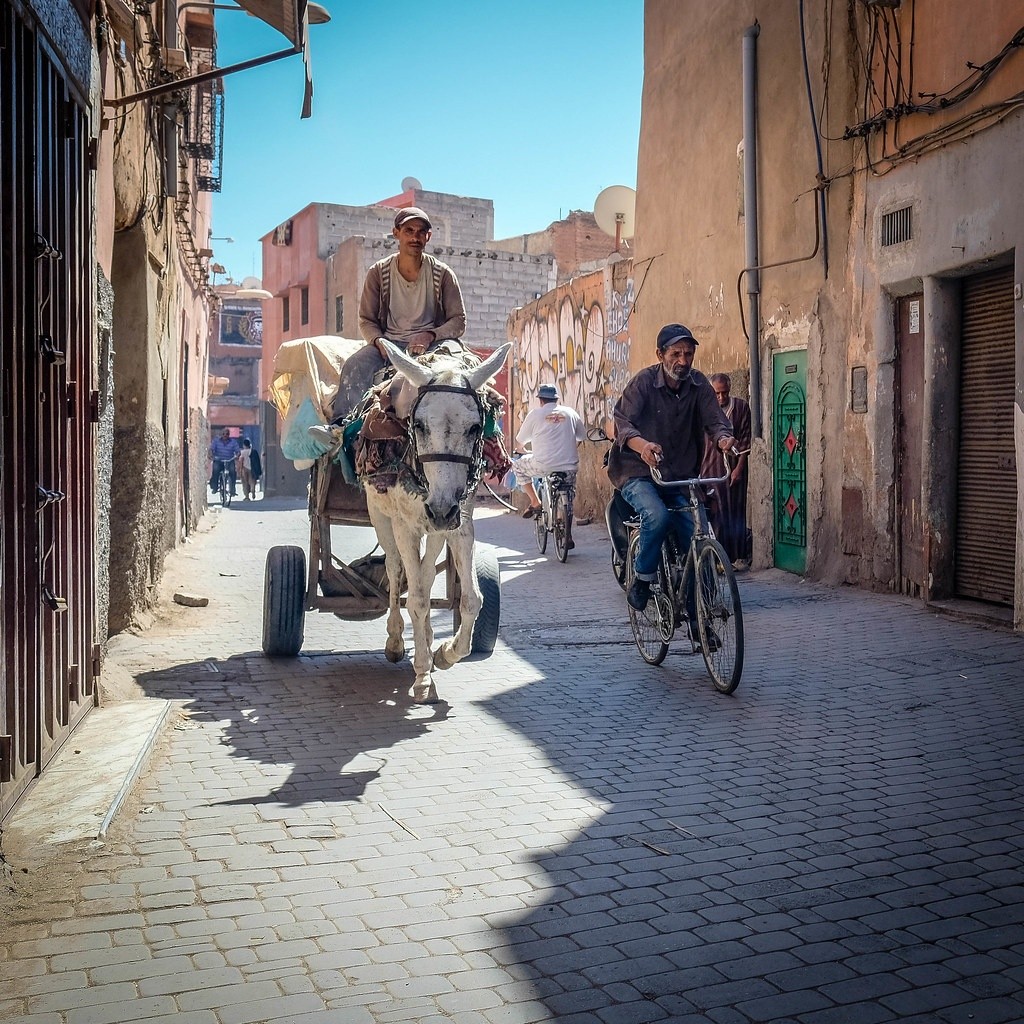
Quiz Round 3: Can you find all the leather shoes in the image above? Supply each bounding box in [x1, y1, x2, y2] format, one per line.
[627, 579, 651, 611]
[686, 627, 725, 650]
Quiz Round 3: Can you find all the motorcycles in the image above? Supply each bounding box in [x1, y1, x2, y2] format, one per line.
[584, 425, 635, 592]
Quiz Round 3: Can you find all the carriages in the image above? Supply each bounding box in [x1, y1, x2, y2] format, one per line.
[257, 338, 513, 705]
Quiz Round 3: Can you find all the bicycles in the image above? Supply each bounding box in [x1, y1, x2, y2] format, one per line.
[212, 455, 240, 508]
[625, 449, 749, 693]
[512, 448, 575, 563]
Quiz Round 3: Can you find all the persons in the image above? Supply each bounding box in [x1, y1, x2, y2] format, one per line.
[238, 439, 262, 500]
[700, 373, 752, 570]
[516, 384, 587, 549]
[607, 324, 739, 648]
[208, 429, 241, 495]
[307, 206, 496, 447]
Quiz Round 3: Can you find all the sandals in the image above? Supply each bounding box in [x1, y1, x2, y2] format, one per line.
[307, 424, 343, 450]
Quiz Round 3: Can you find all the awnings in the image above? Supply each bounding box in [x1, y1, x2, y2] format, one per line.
[105, 0, 313, 119]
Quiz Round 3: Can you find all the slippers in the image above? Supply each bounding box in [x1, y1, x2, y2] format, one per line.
[564, 540, 575, 547]
[522, 503, 542, 518]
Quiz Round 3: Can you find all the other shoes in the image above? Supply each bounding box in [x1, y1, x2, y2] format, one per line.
[242, 498, 251, 501]
[731, 558, 750, 573]
[212, 488, 218, 494]
[229, 492, 238, 496]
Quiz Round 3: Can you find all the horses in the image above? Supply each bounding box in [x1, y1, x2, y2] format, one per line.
[362, 338, 513, 703]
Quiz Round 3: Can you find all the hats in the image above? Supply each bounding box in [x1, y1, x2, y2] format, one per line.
[656, 323, 699, 349]
[536, 384, 561, 399]
[394, 206, 432, 228]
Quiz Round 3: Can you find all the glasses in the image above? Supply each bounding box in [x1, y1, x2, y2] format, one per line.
[222, 432, 229, 436]
